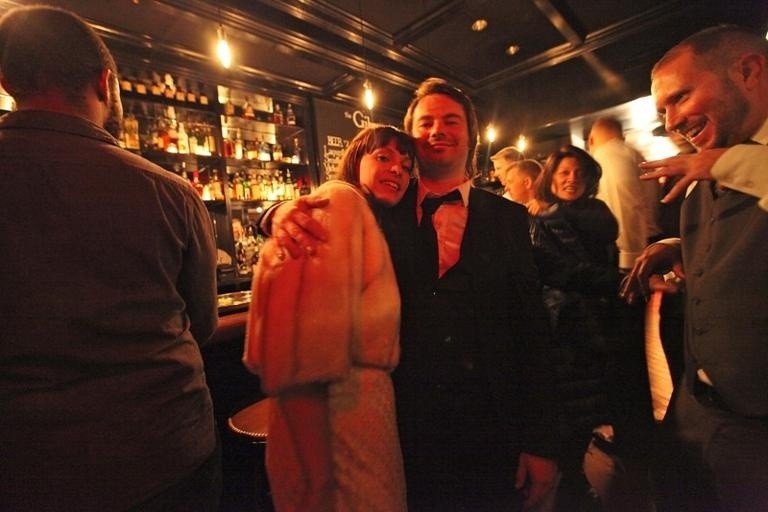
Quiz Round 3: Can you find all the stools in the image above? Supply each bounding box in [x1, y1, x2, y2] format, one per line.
[230, 393, 283, 511]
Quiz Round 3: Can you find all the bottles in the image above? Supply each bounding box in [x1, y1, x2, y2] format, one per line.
[233, 221, 271, 279]
[239, 95, 256, 119]
[271, 102, 283, 125]
[228, 166, 302, 202]
[118, 75, 209, 105]
[118, 108, 217, 157]
[222, 127, 301, 163]
[179, 160, 223, 202]
[284, 102, 297, 126]
[223, 91, 235, 115]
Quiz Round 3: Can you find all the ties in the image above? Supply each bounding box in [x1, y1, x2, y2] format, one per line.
[416, 188, 462, 290]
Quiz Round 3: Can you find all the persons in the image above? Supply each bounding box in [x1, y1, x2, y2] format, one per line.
[488, 144, 526, 206]
[531, 143, 622, 511]
[501, 158, 553, 218]
[255, 75, 563, 512]
[582, 113, 660, 324]
[0, 3, 223, 512]
[240, 123, 416, 512]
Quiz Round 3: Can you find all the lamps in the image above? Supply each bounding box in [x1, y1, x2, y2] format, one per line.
[213, 1, 238, 68]
[359, 1, 378, 110]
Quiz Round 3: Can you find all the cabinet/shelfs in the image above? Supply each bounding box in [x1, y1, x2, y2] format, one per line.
[217, 64, 323, 292]
[95, 34, 237, 297]
